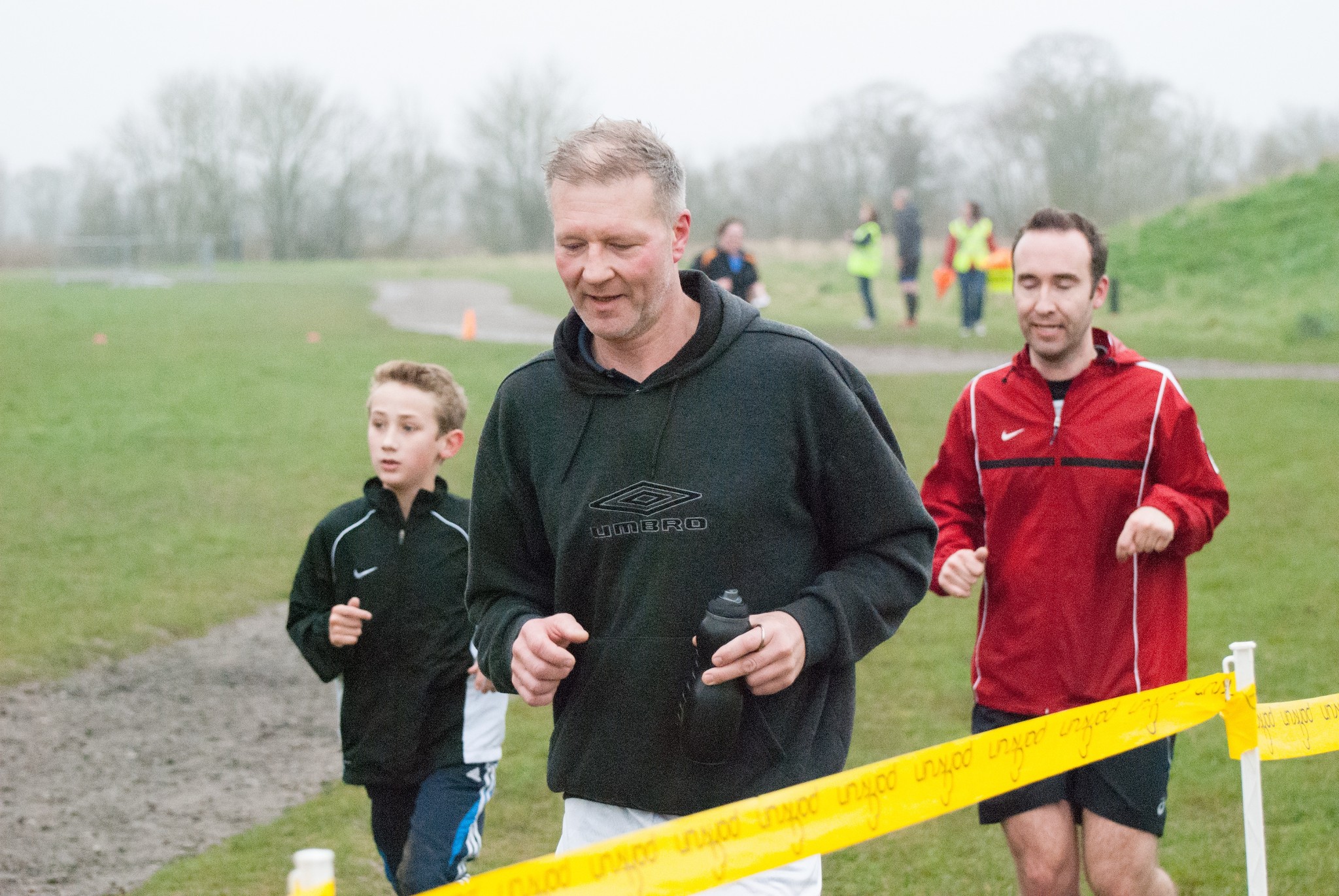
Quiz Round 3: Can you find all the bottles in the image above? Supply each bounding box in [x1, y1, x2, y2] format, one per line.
[678, 588, 754, 765]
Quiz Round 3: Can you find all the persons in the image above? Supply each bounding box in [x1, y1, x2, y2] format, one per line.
[942, 198, 1003, 334]
[918, 206, 1230, 896]
[688, 216, 764, 314]
[842, 204, 881, 328]
[886, 183, 921, 328]
[462, 118, 941, 896]
[284, 360, 515, 895]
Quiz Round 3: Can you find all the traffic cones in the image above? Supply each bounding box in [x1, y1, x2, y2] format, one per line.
[934, 269, 955, 299]
[459, 309, 475, 341]
[982, 247, 1011, 271]
